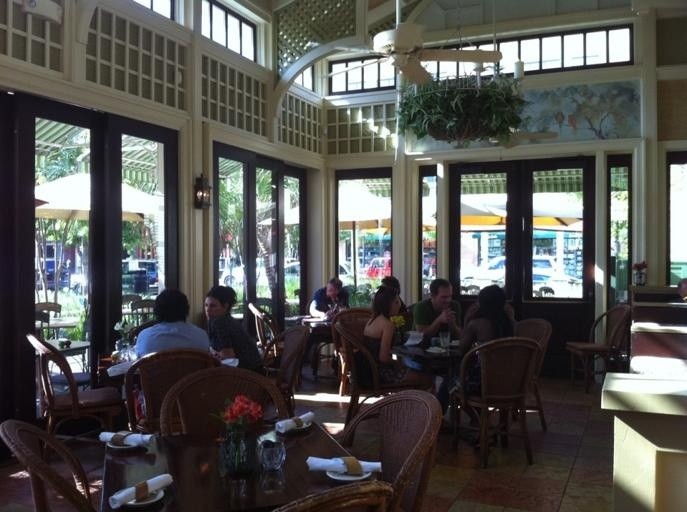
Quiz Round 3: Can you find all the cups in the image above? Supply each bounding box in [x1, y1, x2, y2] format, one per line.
[438, 330, 451, 347]
[256, 440, 288, 470]
[258, 469, 288, 495]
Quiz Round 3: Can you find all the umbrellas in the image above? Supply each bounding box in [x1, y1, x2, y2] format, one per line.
[459, 192, 583, 227]
[35, 173, 157, 223]
[258, 187, 437, 286]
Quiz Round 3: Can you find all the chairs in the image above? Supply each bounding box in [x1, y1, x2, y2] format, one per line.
[566, 304, 631, 393]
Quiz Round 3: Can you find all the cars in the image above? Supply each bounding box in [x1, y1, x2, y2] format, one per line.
[218, 256, 392, 288]
[460, 271, 581, 296]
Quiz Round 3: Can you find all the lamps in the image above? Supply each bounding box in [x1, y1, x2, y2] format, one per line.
[193, 173, 213, 210]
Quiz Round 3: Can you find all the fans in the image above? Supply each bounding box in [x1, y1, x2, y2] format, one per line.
[322, 21, 503, 84]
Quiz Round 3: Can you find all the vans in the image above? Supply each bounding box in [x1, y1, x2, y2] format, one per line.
[70, 259, 158, 295]
[473, 254, 560, 271]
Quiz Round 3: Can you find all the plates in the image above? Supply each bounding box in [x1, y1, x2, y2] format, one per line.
[106, 441, 137, 449]
[325, 471, 373, 482]
[425, 349, 446, 353]
[126, 488, 166, 507]
[295, 421, 312, 430]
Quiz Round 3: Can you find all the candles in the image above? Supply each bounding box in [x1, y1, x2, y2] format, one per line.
[514, 61, 524, 79]
[475, 49, 483, 69]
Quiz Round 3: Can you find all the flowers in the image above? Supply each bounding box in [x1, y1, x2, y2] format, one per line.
[632, 261, 647, 270]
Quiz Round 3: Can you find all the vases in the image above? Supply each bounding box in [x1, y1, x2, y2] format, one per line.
[634, 270, 646, 288]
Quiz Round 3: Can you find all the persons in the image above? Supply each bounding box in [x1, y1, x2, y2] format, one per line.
[449, 285, 517, 448]
[313, 277, 350, 376]
[404, 279, 463, 416]
[359, 286, 434, 393]
[382, 276, 407, 314]
[204, 286, 267, 376]
[134, 289, 209, 419]
